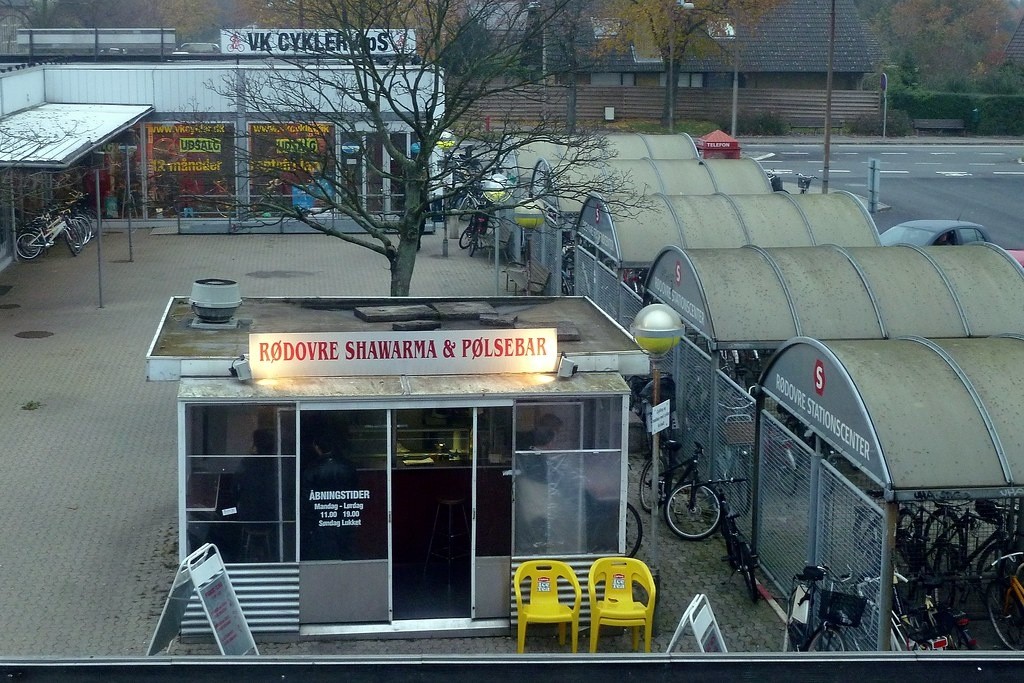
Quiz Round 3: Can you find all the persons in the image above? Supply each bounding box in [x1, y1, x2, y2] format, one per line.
[938, 233, 951, 245]
[220, 429, 362, 561]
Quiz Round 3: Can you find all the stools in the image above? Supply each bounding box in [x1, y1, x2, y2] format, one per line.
[424, 496, 471, 596]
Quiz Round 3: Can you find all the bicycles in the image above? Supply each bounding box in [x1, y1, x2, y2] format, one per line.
[848, 570, 982, 651]
[695, 477, 760, 603]
[693, 339, 847, 493]
[783, 558, 866, 653]
[561, 234, 645, 295]
[16, 189, 98, 260]
[850, 496, 1024, 649]
[459, 201, 488, 257]
[638, 438, 721, 540]
[174, 180, 238, 218]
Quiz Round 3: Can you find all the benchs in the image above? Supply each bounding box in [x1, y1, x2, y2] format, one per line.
[788, 117, 844, 135]
[502, 257, 552, 295]
[481, 222, 511, 262]
[912, 118, 966, 136]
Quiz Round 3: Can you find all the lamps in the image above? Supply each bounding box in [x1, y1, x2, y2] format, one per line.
[558, 360, 578, 377]
[228, 362, 252, 381]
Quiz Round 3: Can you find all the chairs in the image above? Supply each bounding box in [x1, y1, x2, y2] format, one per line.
[588, 557, 657, 654]
[514, 560, 581, 654]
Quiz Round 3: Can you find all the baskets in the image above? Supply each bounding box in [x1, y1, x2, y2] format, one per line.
[818, 587, 867, 628]
[725, 420, 755, 444]
[746, 377, 770, 398]
